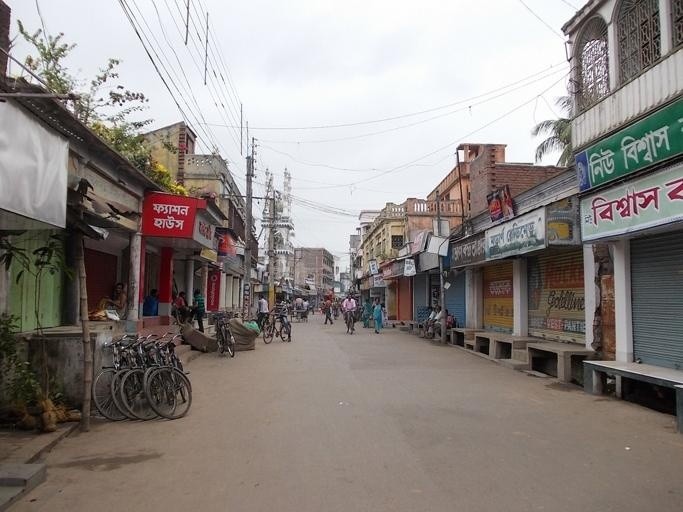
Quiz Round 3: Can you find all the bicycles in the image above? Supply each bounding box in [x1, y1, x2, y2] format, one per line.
[259, 313, 292, 344]
[246, 314, 272, 338]
[342, 308, 357, 334]
[211, 312, 236, 359]
[89, 332, 192, 422]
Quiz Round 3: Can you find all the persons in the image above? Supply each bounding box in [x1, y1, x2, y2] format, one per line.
[143, 287, 205, 333]
[418, 305, 454, 338]
[104, 283, 128, 320]
[257, 294, 269, 329]
[295, 294, 387, 335]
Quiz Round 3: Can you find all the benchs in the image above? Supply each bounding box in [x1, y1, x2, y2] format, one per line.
[406, 319, 682, 429]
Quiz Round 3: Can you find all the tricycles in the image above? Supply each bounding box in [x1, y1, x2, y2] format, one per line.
[291, 306, 308, 323]
